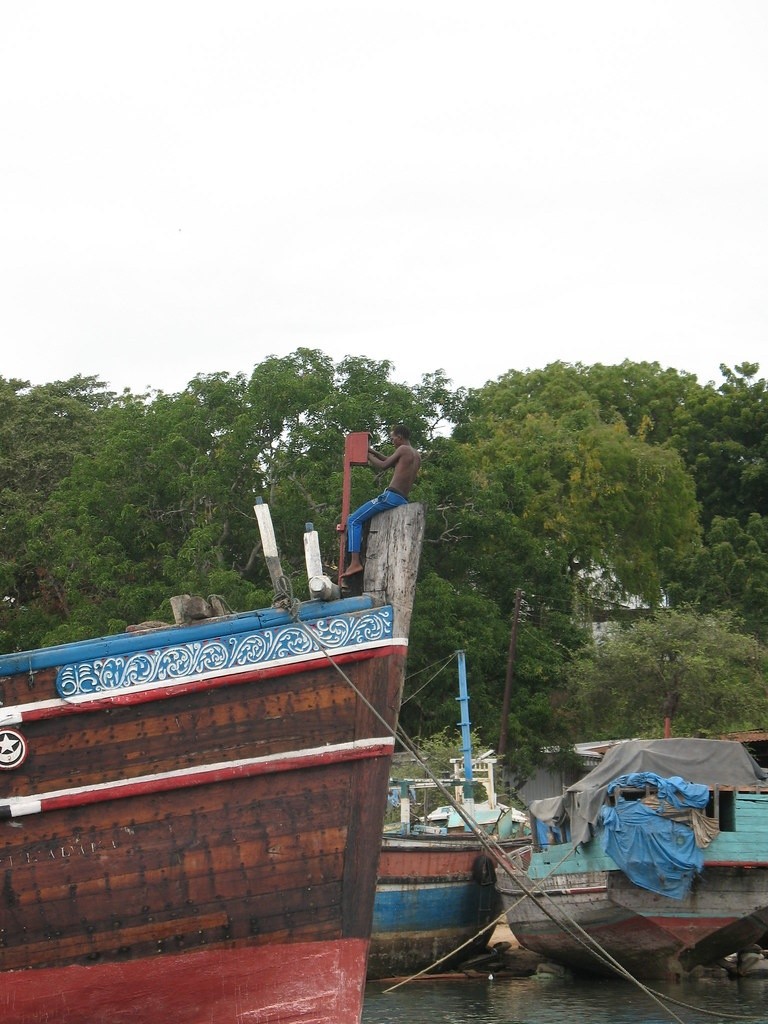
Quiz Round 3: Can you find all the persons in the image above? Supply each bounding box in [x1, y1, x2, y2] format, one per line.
[341, 424, 421, 577]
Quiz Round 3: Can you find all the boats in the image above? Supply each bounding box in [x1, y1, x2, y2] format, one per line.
[0, 596, 409, 1023]
[493, 739, 768, 980]
[366, 652, 556, 979]
